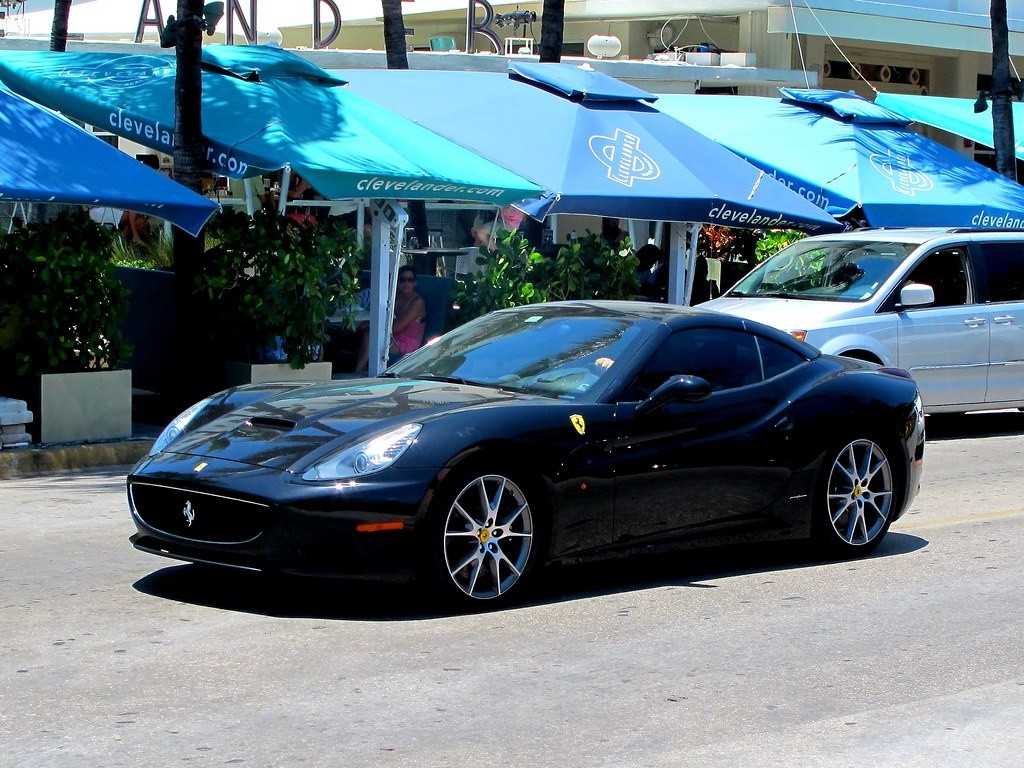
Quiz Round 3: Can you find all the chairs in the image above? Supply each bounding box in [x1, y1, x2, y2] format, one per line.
[691, 337, 737, 393]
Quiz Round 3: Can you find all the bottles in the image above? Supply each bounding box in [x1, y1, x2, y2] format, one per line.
[571, 230, 577, 244]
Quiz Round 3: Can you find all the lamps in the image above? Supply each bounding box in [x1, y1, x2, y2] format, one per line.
[159, 14, 202, 48]
[973, 89, 1013, 114]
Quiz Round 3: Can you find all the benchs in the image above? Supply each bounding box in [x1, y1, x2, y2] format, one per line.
[330, 269, 452, 366]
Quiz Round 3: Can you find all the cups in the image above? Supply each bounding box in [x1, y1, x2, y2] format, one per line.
[274, 182, 279, 189]
[264, 179, 270, 188]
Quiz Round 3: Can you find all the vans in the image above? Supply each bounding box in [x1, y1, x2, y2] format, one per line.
[692, 225, 1024, 414]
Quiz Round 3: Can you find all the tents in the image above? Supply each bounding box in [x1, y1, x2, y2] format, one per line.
[0, 45, 1024, 375]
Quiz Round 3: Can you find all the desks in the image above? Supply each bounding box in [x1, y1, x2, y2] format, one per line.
[325, 309, 397, 323]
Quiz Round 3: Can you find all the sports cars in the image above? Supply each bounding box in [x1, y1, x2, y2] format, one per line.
[124, 301, 927, 610]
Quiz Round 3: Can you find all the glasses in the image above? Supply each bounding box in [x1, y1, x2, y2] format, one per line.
[398, 277, 416, 284]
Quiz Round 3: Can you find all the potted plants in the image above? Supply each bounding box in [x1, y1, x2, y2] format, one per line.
[8, 213, 149, 445]
[202, 211, 332, 384]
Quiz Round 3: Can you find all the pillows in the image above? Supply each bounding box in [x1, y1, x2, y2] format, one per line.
[354, 288, 371, 312]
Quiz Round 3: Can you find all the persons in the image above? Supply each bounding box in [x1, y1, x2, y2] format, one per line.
[476, 208, 524, 252]
[288, 171, 318, 226]
[633, 244, 659, 284]
[88, 206, 148, 248]
[691, 255, 720, 306]
[354, 265, 427, 374]
